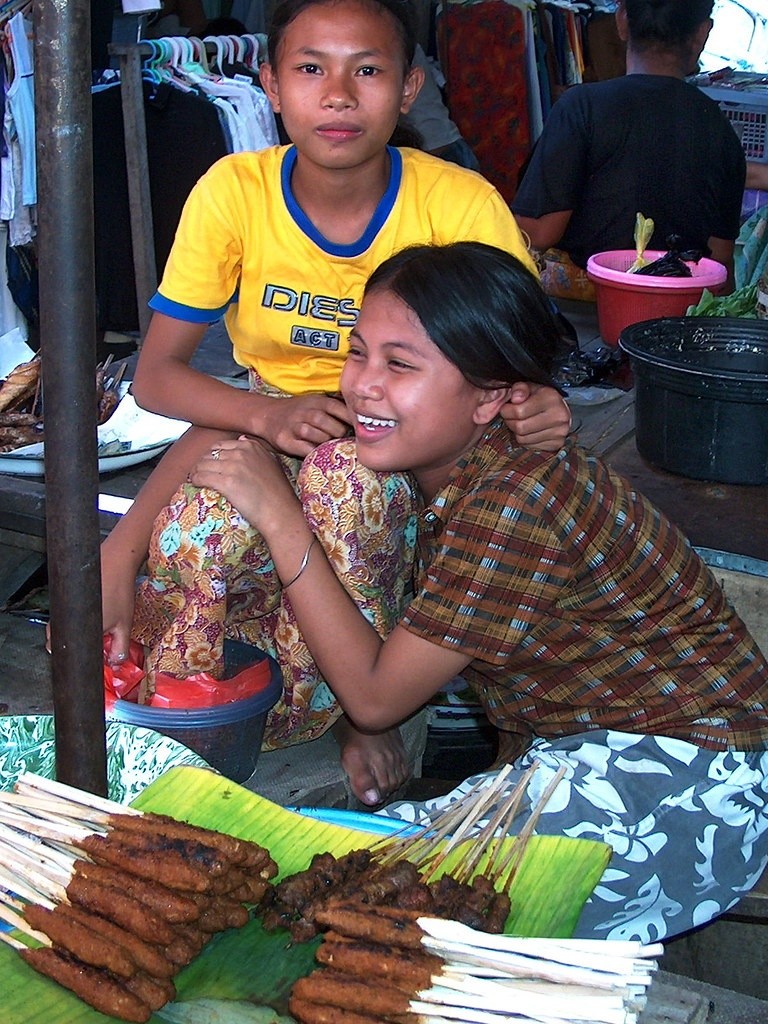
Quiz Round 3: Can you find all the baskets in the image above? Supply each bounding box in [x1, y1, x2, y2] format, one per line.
[105, 636, 283, 787]
[588, 249, 727, 347]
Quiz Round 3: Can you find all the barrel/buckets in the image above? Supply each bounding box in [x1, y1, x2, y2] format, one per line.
[618, 316, 768, 484]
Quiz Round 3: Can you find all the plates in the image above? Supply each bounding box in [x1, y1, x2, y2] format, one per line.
[0, 377, 173, 476]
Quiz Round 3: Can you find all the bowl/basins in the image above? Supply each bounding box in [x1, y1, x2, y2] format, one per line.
[587, 250, 727, 346]
[104, 639, 282, 785]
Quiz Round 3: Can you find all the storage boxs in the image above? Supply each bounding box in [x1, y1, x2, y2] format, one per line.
[685, 70, 768, 165]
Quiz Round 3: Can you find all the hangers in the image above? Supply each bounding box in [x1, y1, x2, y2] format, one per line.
[106, 32, 269, 96]
[0, 0, 33, 56]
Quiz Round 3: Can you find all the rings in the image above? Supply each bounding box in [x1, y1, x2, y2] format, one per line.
[211, 448, 221, 460]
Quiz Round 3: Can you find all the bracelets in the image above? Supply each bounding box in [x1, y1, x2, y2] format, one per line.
[280, 529, 317, 596]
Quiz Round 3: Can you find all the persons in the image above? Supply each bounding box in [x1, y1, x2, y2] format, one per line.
[131, 0, 574, 806]
[45, 231, 768, 943]
[745, 161, 768, 189]
[405, 41, 480, 172]
[511, 0, 746, 263]
[147, 0, 250, 39]
